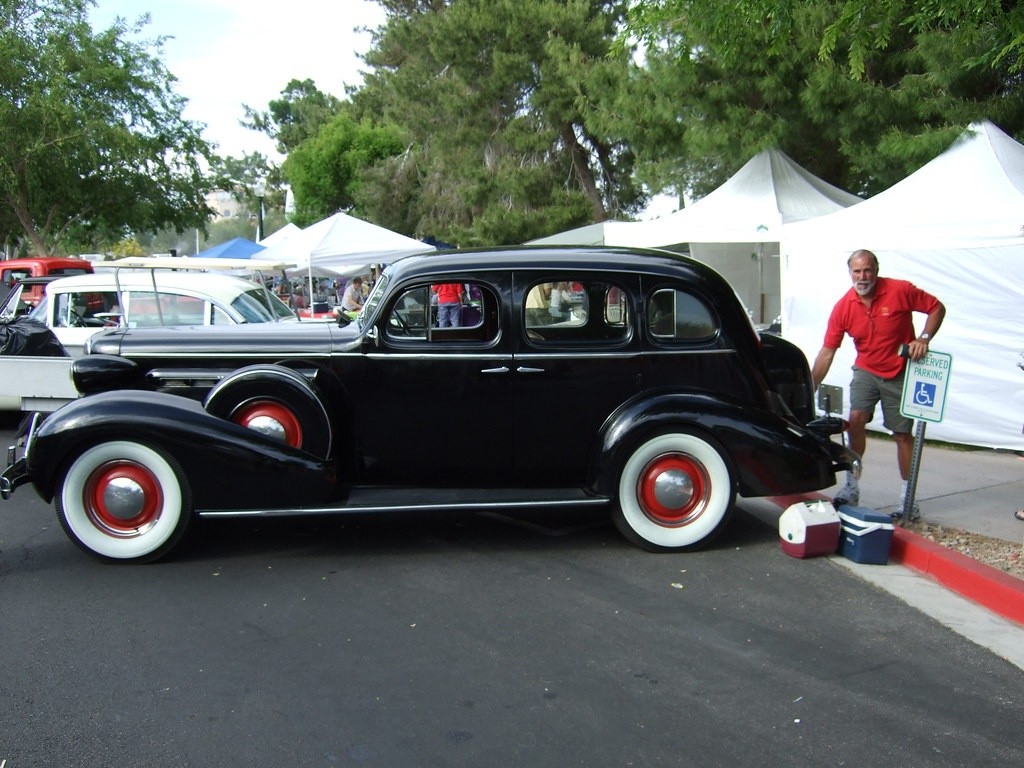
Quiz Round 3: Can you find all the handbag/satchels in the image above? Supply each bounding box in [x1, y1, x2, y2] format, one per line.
[559, 301, 569, 312]
[0, 314, 70, 356]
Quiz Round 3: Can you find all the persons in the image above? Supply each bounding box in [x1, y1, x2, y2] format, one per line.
[103, 305, 122, 327]
[264, 275, 481, 328]
[526, 279, 626, 327]
[812, 249, 946, 523]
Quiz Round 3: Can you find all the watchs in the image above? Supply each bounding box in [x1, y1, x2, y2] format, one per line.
[920, 333, 930, 342]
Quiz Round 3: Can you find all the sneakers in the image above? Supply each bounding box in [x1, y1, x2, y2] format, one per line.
[834, 482, 858, 507]
[890, 503, 921, 521]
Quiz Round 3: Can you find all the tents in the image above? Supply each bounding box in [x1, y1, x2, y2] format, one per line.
[603, 112, 1024, 453]
[187, 212, 457, 318]
[519, 219, 625, 246]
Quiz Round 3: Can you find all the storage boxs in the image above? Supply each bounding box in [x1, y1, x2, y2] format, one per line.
[779, 500, 894, 565]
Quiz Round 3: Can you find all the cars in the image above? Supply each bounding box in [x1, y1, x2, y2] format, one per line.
[0, 243, 861, 567]
[0, 254, 299, 412]
